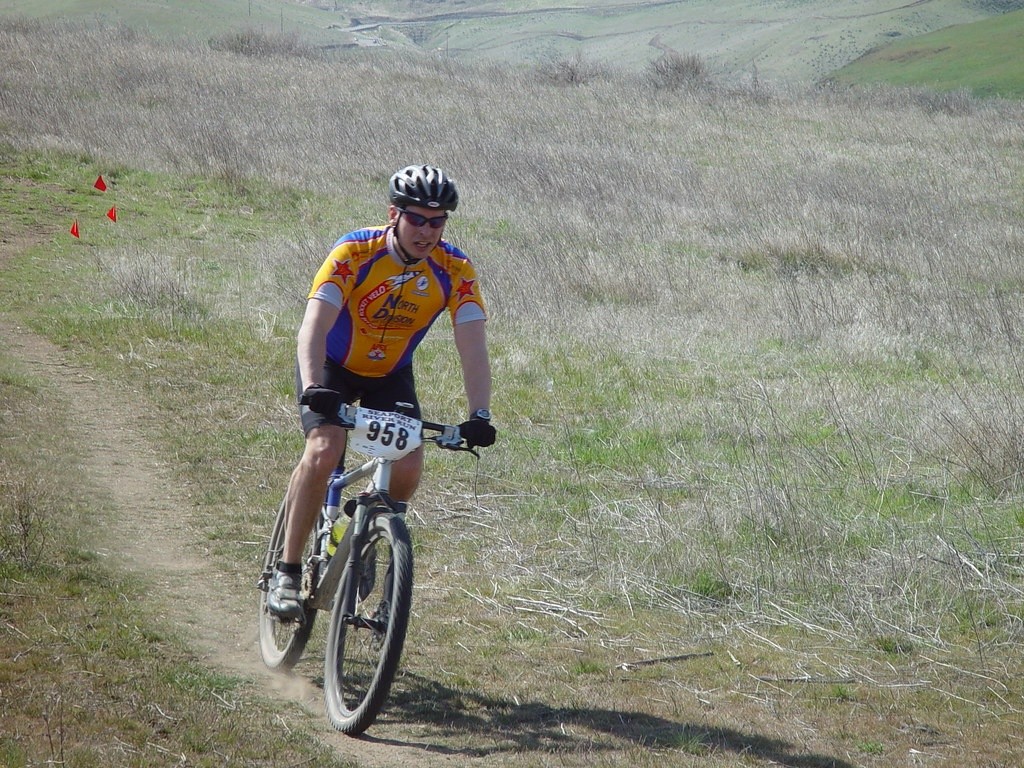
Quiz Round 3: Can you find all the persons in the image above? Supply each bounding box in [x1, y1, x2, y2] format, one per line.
[268, 164, 497, 633]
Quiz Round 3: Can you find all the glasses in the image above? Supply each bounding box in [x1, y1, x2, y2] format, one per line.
[396, 206, 448, 228]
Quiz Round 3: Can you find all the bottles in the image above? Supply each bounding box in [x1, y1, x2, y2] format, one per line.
[325, 499, 356, 556]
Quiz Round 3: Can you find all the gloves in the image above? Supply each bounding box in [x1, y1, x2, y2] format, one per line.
[303, 384, 341, 419]
[457, 420, 495, 448]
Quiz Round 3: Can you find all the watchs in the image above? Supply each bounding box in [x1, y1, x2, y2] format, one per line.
[470, 408, 491, 421]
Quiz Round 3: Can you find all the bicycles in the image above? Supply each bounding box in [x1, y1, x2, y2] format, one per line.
[255, 391, 482, 737]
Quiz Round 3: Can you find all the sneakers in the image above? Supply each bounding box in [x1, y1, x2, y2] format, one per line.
[266, 560, 301, 617]
[359, 548, 377, 601]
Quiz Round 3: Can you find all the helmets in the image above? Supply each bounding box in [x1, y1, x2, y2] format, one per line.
[390, 164, 458, 212]
